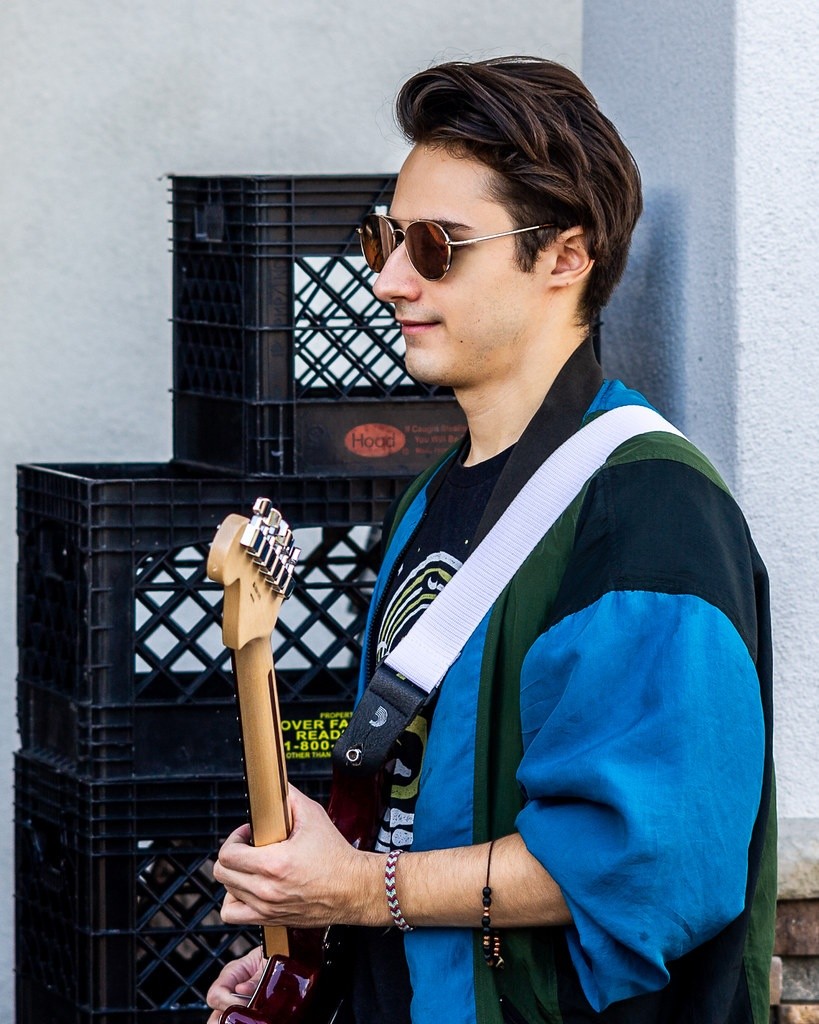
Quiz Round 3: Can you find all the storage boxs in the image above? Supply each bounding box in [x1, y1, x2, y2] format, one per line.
[166, 172, 604, 484]
[10, 751, 412, 1024]
[11, 460, 412, 779]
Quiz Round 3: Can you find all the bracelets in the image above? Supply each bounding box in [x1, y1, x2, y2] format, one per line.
[481, 839, 505, 970]
[385, 849, 415, 934]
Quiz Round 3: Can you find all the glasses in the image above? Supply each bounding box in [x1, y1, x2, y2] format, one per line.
[356, 214, 555, 282]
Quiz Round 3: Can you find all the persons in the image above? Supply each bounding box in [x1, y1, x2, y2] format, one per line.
[205, 54, 778, 1023]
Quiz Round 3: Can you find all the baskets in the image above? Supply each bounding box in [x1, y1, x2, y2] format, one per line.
[18, 460, 418, 781]
[164, 173, 473, 476]
[16, 786, 333, 1024]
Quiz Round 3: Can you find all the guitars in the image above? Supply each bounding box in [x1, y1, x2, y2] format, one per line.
[204, 493, 393, 1024]
